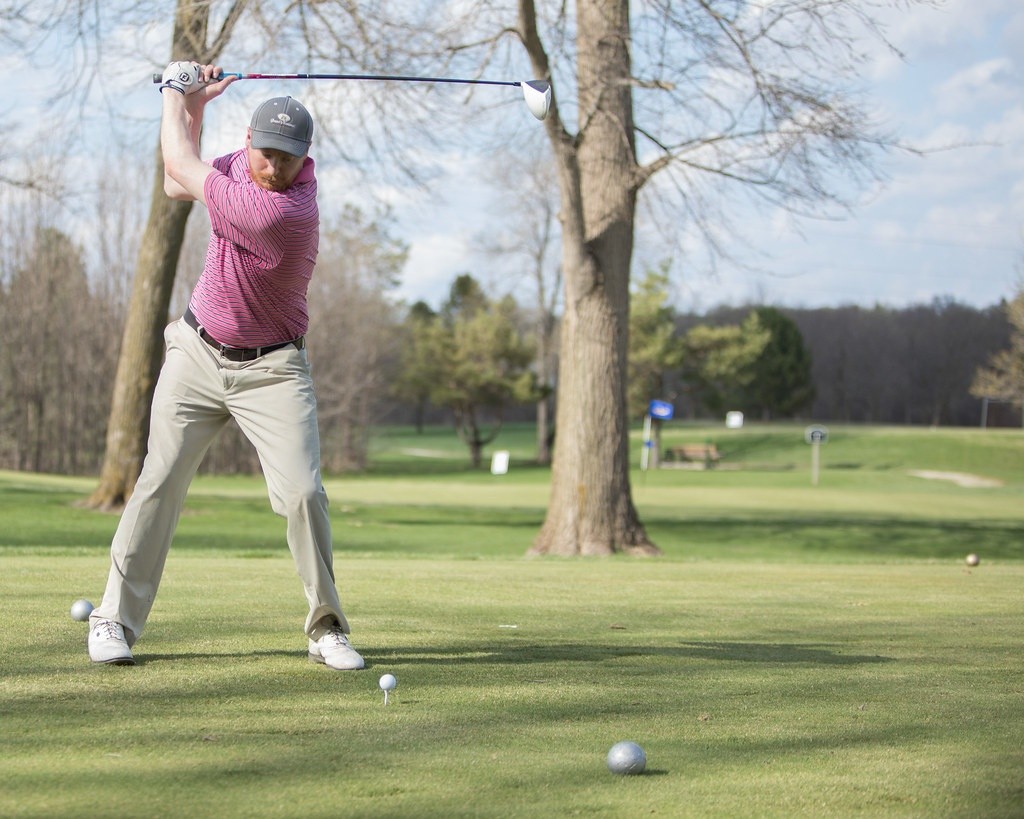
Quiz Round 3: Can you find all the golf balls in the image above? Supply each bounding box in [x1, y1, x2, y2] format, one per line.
[379, 674, 397, 688]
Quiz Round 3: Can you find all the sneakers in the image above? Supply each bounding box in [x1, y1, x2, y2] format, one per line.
[88, 621, 134, 666]
[309, 626, 365, 669]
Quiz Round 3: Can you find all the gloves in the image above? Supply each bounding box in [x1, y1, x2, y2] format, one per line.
[158, 61, 218, 95]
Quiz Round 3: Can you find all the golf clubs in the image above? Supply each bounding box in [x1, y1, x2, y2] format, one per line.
[153, 72, 554, 122]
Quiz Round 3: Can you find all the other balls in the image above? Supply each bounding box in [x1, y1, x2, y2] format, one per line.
[71, 600, 94, 621]
[606, 741, 646, 776]
[966, 553, 979, 566]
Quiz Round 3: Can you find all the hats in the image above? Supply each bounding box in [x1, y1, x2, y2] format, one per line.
[250, 95, 313, 158]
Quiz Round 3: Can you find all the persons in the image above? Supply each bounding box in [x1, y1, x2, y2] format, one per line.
[88, 60, 365, 668]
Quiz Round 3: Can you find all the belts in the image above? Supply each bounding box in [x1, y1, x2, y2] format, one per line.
[183, 306, 305, 362]
